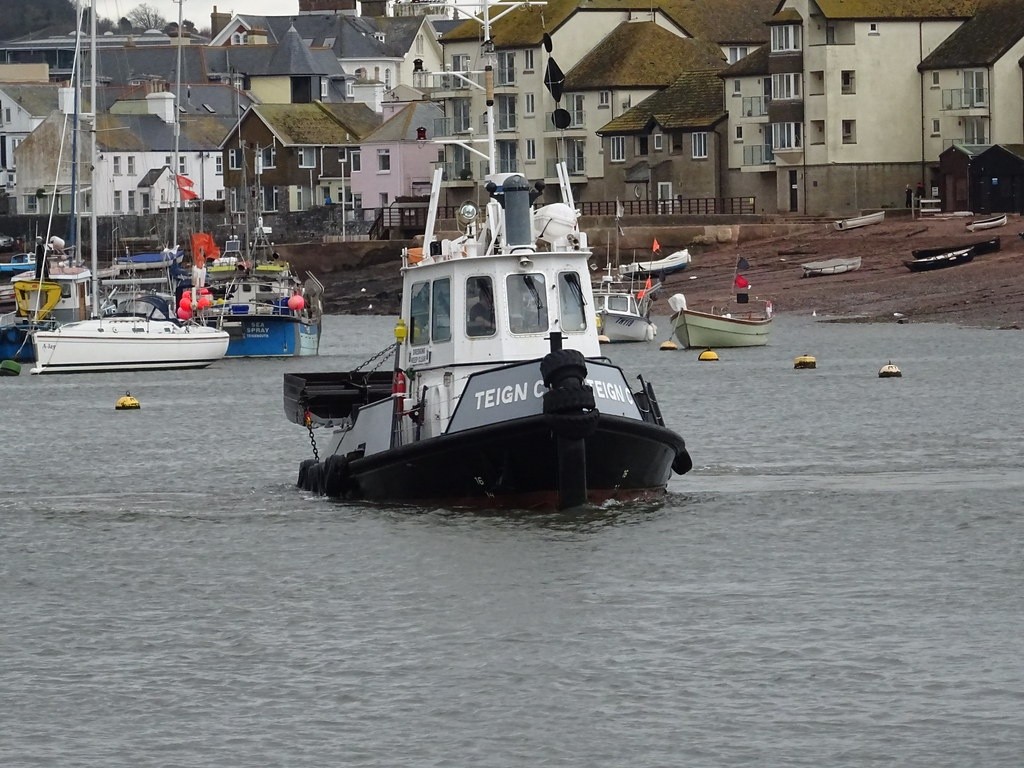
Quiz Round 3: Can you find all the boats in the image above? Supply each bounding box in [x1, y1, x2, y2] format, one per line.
[283, 0, 694, 518]
[800, 256, 863, 279]
[901, 243, 976, 273]
[666, 292, 776, 350]
[833, 210, 886, 231]
[910, 235, 1001, 259]
[965, 212, 1008, 232]
[590, 211, 663, 345]
[618, 248, 692, 281]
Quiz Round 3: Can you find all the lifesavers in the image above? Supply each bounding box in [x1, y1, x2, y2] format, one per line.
[671, 449, 693, 475]
[392, 372, 405, 420]
[296, 453, 346, 496]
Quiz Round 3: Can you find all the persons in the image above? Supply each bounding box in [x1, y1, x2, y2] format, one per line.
[906, 184, 912, 208]
[914, 183, 926, 208]
[471, 287, 495, 328]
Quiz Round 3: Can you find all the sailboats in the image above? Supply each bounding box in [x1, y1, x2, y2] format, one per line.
[0, 0, 326, 379]
